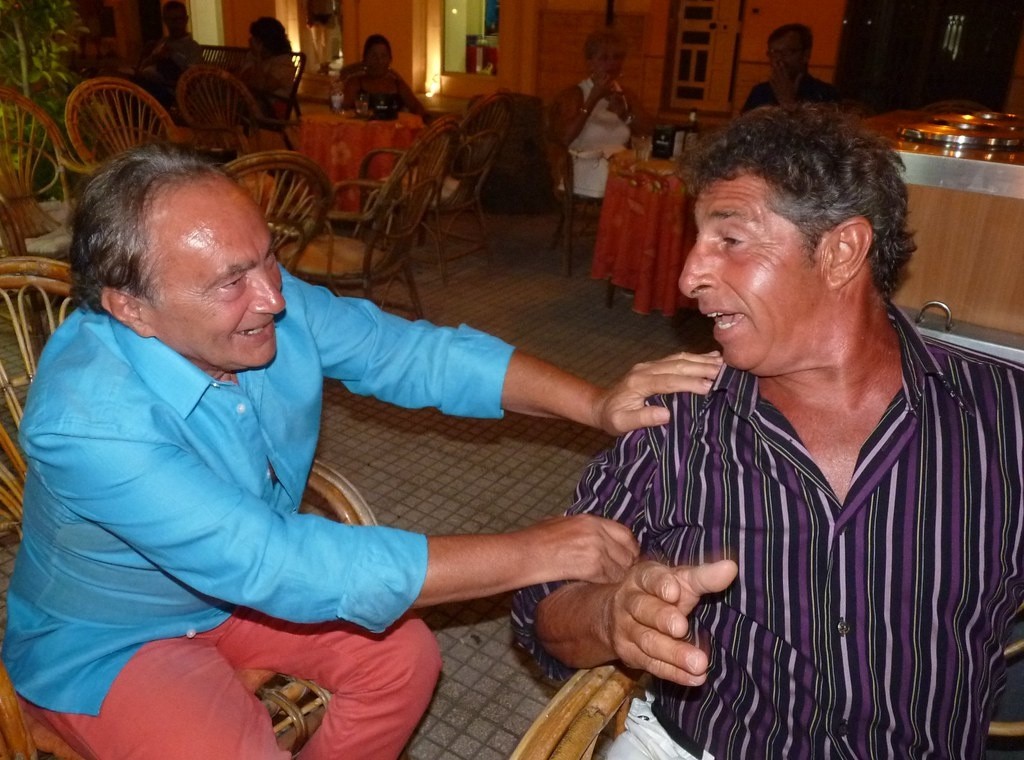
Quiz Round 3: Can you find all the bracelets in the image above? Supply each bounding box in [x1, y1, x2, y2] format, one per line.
[580, 108, 587, 113]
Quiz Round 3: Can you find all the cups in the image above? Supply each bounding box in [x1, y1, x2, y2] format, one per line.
[353, 93, 369, 116]
[631, 133, 652, 163]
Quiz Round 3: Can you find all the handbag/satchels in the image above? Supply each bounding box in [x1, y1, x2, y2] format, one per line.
[556, 148, 618, 199]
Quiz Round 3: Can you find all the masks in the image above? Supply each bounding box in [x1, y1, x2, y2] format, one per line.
[367, 54, 391, 73]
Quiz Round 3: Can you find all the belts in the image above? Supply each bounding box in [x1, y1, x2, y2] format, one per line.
[651, 699, 703, 760]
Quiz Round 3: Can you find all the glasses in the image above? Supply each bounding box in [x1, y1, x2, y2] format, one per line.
[767, 47, 805, 61]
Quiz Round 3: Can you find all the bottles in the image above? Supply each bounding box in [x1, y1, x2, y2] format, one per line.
[683, 107, 699, 152]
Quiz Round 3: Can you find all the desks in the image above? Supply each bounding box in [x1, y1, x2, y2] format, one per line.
[590, 150, 701, 319]
[285, 105, 426, 215]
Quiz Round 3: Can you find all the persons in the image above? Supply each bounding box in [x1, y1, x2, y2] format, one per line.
[329, 34, 433, 126]
[0, 141, 724, 760]
[740, 22, 836, 116]
[511, 105, 1024, 760]
[235, 16, 295, 131]
[553, 30, 635, 206]
[137, 0, 202, 113]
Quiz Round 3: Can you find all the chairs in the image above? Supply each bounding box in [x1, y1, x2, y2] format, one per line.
[1, 45, 1024, 760]
